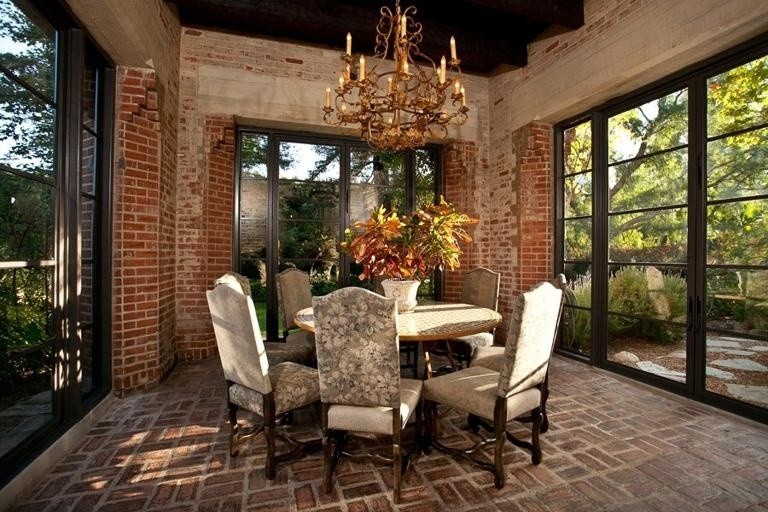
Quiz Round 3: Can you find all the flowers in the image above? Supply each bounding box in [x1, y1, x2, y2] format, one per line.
[340, 193, 480, 281]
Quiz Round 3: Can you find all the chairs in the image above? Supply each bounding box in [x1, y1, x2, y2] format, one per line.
[422, 267, 567, 490]
[203, 266, 322, 480]
[312, 287, 423, 503]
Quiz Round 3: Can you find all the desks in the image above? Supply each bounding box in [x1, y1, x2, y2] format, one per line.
[294, 300, 503, 457]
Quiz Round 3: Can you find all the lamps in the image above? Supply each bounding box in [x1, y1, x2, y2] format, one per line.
[321, 0, 472, 156]
